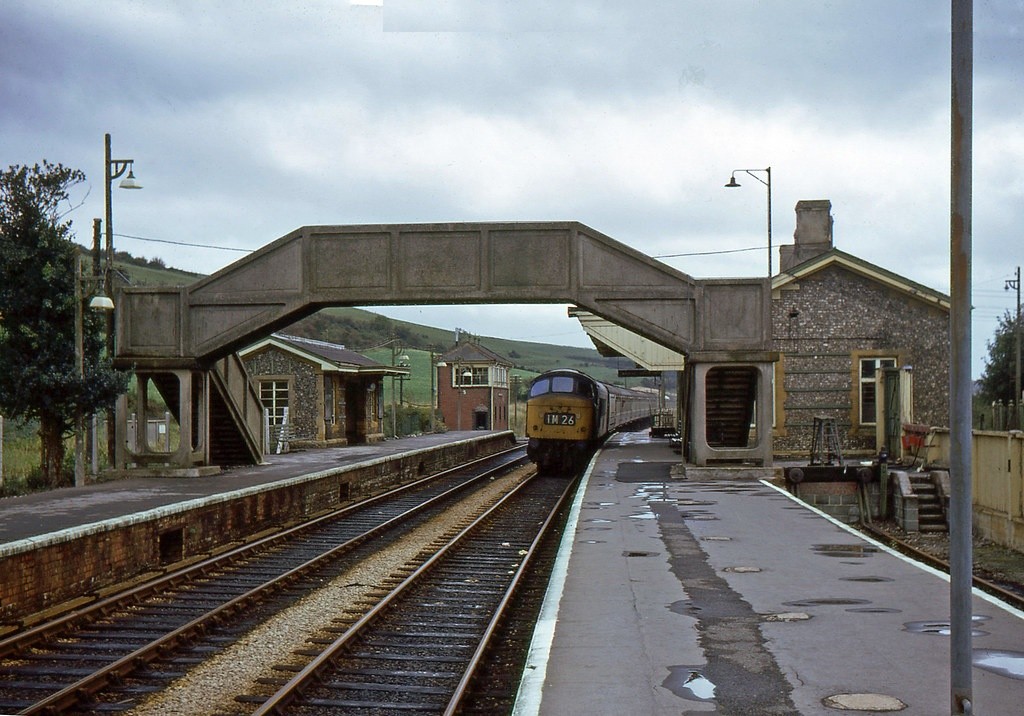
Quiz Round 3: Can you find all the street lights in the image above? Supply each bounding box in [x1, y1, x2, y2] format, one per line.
[1004, 266, 1020, 428]
[74, 252, 114, 487]
[725, 167, 774, 277]
[105, 133, 144, 469]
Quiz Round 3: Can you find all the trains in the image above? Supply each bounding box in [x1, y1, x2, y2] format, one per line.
[525, 368, 670, 477]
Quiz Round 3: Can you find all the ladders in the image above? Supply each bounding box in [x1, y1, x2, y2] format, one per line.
[811, 414, 845, 465]
